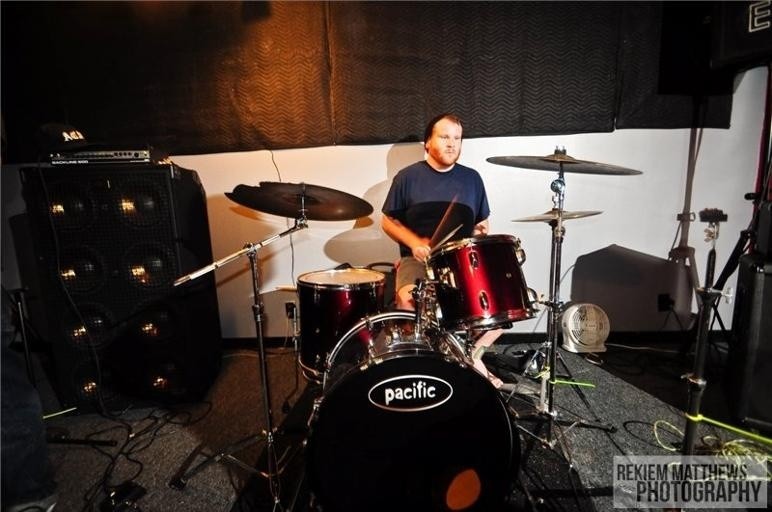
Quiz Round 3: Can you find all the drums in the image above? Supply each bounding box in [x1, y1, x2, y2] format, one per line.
[298, 269, 386, 382]
[301, 312, 522, 512]
[426, 234, 536, 333]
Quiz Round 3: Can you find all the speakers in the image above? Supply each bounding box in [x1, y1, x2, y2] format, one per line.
[721, 253, 772, 438]
[19, 164, 223, 403]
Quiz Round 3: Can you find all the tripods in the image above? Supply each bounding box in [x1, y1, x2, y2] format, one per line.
[170, 251, 310, 512]
[505, 177, 634, 486]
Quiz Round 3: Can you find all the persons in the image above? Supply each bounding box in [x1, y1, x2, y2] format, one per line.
[381, 114, 506, 391]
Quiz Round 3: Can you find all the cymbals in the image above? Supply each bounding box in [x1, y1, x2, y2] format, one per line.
[224, 182, 373, 221]
[511, 212, 601, 222]
[487, 156, 643, 175]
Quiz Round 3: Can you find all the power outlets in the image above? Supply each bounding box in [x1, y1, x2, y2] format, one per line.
[286, 303, 297, 320]
[656, 294, 675, 313]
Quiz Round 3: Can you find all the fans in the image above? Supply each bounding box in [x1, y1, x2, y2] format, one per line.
[562, 301, 611, 353]
